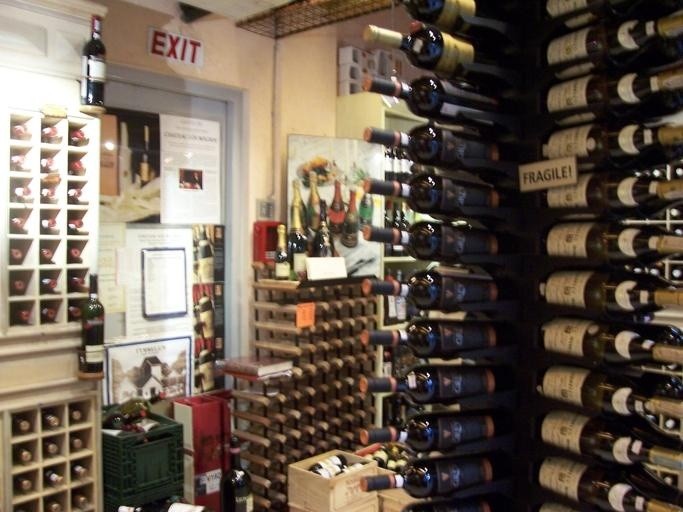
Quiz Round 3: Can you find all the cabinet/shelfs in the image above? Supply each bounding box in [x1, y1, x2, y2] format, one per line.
[220, 252, 383, 509]
[0, 106, 103, 511]
[373, 0, 683, 512]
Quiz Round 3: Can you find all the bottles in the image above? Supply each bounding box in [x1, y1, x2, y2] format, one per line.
[275, 224, 290, 279]
[400, 0, 486, 37]
[541, 219, 683, 266]
[541, 68, 683, 125]
[531, 403, 683, 473]
[359, 408, 494, 453]
[328, 180, 345, 234]
[198, 292, 215, 339]
[199, 338, 216, 392]
[361, 318, 497, 359]
[359, 452, 496, 499]
[530, 450, 683, 512]
[362, 170, 500, 218]
[371, 444, 410, 472]
[361, 74, 502, 131]
[80, 14, 106, 106]
[198, 224, 214, 284]
[102, 411, 146, 429]
[359, 364, 496, 403]
[361, 268, 498, 312]
[540, 269, 682, 314]
[139, 125, 154, 190]
[290, 179, 306, 226]
[81, 273, 104, 373]
[306, 171, 321, 230]
[363, 123, 500, 177]
[289, 203, 307, 273]
[103, 390, 166, 421]
[541, 10, 683, 82]
[219, 436, 254, 512]
[364, 24, 489, 79]
[308, 454, 369, 478]
[539, 170, 683, 214]
[362, 220, 500, 263]
[532, 360, 683, 420]
[541, 123, 683, 162]
[536, 316, 683, 365]
[358, 192, 373, 232]
[314, 200, 334, 258]
[340, 189, 358, 247]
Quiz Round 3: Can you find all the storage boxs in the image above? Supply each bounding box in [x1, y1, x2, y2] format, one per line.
[287, 449, 377, 511]
[102, 410, 183, 508]
[356, 441, 431, 512]
